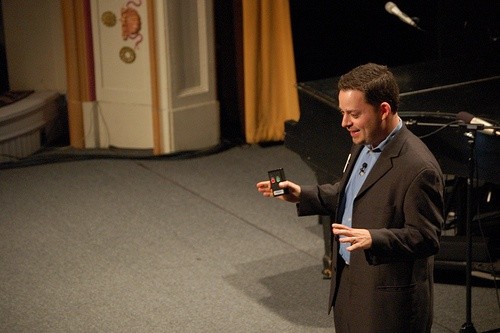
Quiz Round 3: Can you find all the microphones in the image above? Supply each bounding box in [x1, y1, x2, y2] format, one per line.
[385, 1, 422, 32]
[455, 111, 497, 136]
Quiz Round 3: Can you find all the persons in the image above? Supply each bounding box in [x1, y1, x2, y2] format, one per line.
[256, 62, 441, 333]
